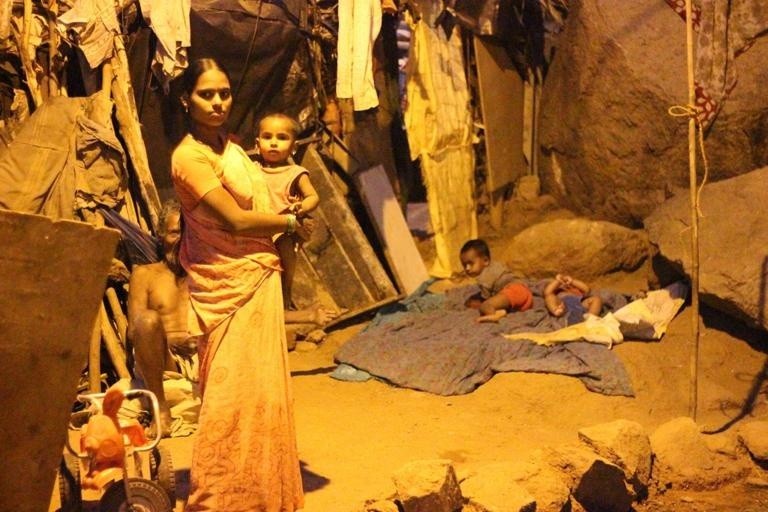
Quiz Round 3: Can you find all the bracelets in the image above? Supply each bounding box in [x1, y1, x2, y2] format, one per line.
[284, 214, 298, 236]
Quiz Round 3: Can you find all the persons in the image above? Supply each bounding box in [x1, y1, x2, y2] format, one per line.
[253, 112, 320, 310]
[281, 306, 336, 327]
[168, 56, 316, 512]
[458, 238, 532, 323]
[542, 274, 603, 327]
[125, 198, 202, 439]
